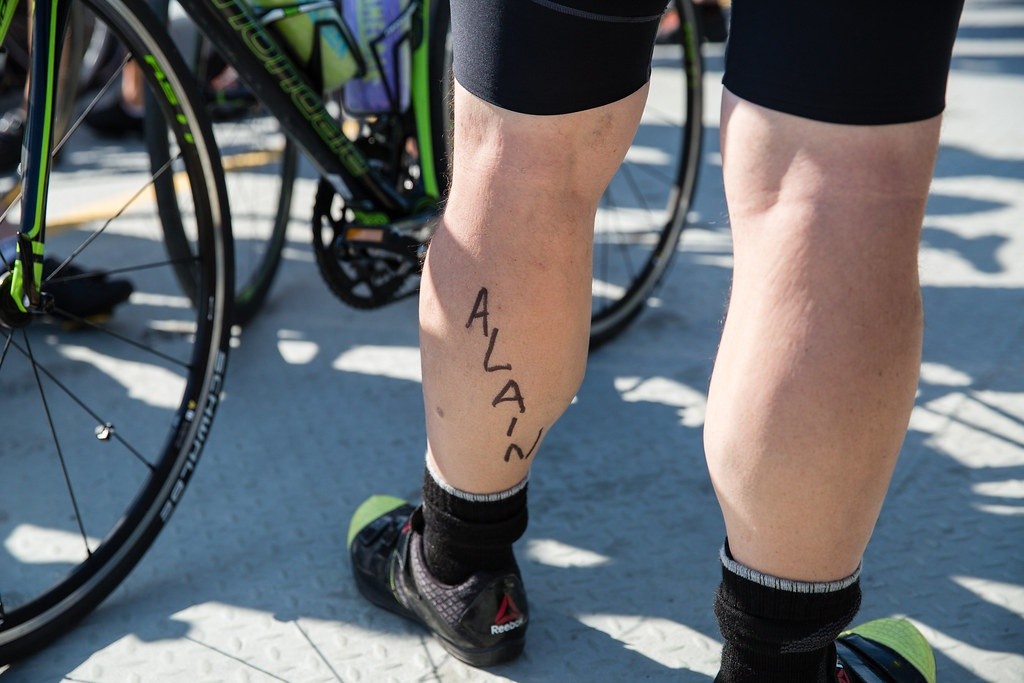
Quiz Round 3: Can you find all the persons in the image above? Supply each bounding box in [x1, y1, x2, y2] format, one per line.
[346, 0, 963, 683]
[0, 1, 260, 319]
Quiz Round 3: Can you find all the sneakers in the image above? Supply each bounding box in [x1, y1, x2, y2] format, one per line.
[831, 615, 936, 683]
[346, 492, 529, 668]
[0, 254, 132, 325]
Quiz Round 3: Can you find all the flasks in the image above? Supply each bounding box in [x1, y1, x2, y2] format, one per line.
[244, 0, 362, 98]
[335, 0, 414, 119]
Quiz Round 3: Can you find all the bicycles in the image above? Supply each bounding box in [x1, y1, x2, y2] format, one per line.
[2, 0, 703, 644]
